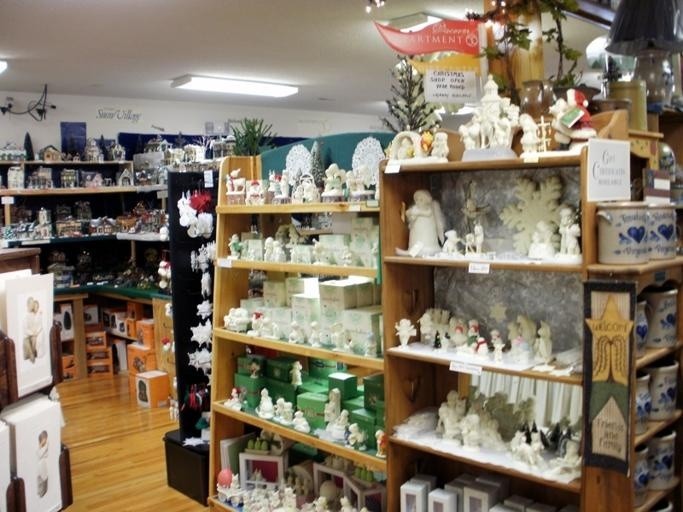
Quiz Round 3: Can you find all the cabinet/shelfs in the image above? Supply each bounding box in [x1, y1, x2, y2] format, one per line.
[378, 108, 630, 512]
[585, 259, 683, 512]
[208, 131, 396, 512]
[164, 166, 219, 498]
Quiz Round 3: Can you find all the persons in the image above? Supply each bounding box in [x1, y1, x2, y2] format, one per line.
[2, 162, 387, 511]
[387, 114, 582, 484]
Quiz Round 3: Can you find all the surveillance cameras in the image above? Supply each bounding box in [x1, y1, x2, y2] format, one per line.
[5, 97, 14, 108]
[36, 101, 56, 111]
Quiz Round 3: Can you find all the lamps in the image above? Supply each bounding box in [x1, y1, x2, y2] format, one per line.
[0, 84, 57, 122]
[387, 15, 443, 33]
[171, 76, 299, 98]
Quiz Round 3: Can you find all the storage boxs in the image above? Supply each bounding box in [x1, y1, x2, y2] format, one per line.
[153, 298, 178, 399]
